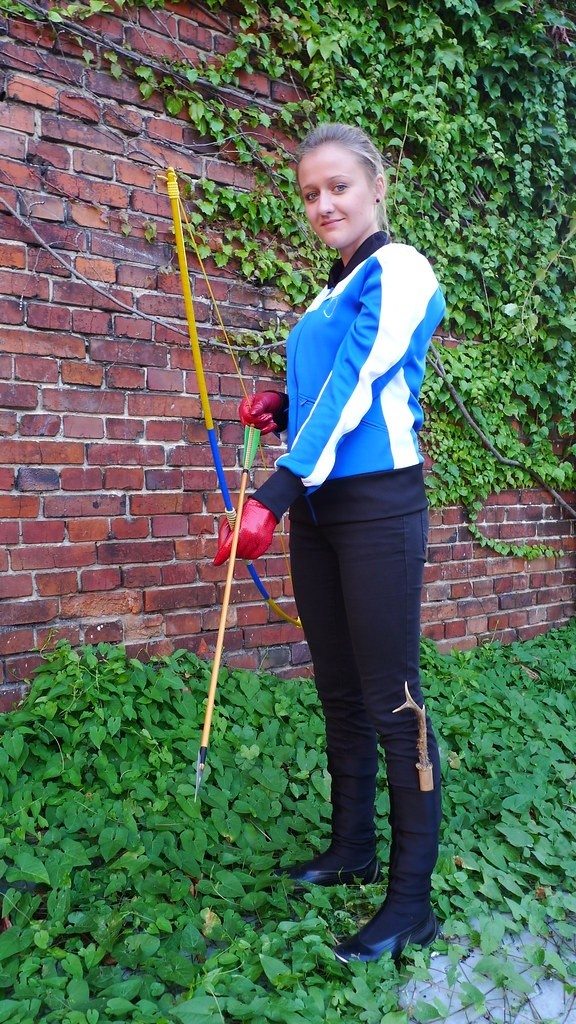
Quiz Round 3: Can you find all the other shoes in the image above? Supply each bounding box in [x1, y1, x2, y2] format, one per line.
[274, 853, 381, 892]
[333, 904, 439, 968]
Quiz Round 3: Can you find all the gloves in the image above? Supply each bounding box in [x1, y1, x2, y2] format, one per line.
[239, 391, 281, 437]
[212, 497, 277, 567]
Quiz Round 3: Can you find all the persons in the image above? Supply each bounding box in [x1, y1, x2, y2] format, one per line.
[212, 123, 446, 969]
